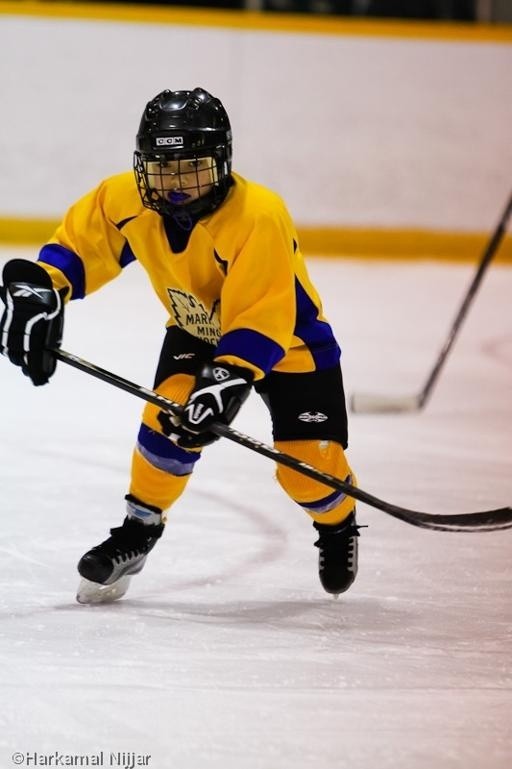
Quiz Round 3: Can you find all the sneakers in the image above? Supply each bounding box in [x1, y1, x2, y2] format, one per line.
[311, 507, 359, 595]
[77, 494, 166, 586]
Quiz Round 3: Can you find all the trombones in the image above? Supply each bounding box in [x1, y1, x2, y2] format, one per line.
[57, 348, 512, 532]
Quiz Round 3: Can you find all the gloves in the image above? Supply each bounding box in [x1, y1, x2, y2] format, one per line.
[0, 253, 70, 387]
[154, 359, 256, 449]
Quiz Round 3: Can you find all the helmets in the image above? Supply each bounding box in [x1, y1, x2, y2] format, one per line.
[131, 86, 233, 223]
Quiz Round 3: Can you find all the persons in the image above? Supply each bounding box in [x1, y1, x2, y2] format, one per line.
[0, 88, 360, 595]
